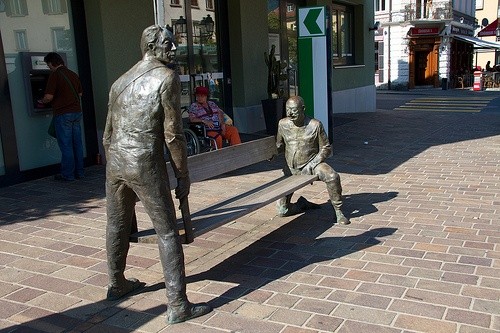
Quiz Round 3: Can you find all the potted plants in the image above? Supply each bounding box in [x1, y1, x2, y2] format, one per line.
[261, 44, 286, 138]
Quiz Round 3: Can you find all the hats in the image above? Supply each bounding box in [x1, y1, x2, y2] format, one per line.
[194, 86, 209, 97]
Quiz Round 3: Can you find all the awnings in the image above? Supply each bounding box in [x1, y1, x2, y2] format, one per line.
[453, 34, 500, 49]
[406, 25, 449, 36]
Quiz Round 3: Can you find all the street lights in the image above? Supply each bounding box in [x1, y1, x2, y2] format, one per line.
[175, 13, 215, 88]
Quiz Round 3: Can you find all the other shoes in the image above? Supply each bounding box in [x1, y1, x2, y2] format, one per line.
[74, 174, 84, 180]
[55, 175, 74, 184]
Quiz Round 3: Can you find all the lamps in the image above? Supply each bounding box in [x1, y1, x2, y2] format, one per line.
[442, 32, 448, 50]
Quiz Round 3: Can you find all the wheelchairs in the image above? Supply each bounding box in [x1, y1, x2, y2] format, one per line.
[180, 105, 222, 157]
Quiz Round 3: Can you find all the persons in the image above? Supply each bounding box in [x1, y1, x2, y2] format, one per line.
[102, 22, 212, 325]
[188, 86, 242, 149]
[485, 60, 491, 71]
[269, 95, 353, 225]
[35, 52, 87, 182]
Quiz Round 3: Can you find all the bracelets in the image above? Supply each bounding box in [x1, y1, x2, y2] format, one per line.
[201, 119, 205, 122]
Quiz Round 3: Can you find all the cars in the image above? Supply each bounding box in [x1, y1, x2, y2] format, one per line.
[182, 89, 189, 95]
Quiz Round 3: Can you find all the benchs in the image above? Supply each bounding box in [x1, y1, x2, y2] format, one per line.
[131, 136, 319, 244]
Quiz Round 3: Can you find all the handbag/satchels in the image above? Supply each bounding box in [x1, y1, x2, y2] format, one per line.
[48, 118, 57, 139]
